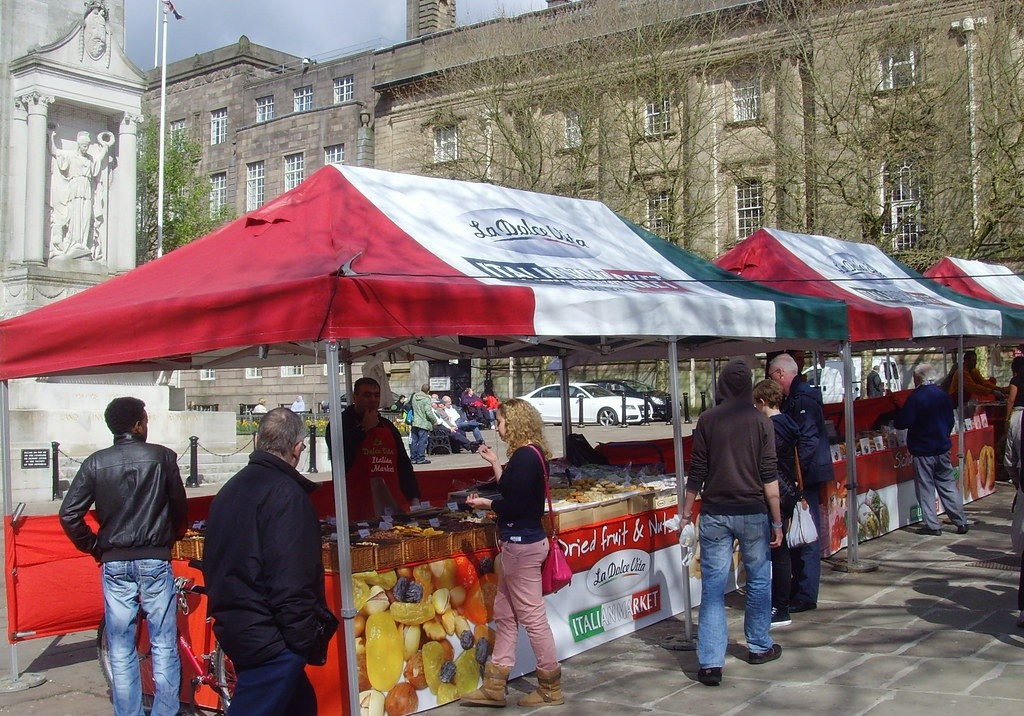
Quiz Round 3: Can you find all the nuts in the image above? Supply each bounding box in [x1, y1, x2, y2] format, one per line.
[347, 558, 499, 716]
[963, 444, 996, 500]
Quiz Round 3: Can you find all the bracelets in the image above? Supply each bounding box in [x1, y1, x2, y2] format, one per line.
[771, 520, 782, 528]
[682, 511, 693, 520]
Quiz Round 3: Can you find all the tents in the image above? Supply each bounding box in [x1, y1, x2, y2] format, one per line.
[886, 256, 1024, 393]
[0, 162, 853, 716]
[546, 227, 1024, 568]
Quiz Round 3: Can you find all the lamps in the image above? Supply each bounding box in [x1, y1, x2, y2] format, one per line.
[302, 58, 310, 68]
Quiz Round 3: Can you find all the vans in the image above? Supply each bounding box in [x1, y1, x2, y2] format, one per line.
[801, 356, 902, 405]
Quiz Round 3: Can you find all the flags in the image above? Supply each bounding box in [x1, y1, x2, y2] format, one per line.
[168, 2, 183, 20]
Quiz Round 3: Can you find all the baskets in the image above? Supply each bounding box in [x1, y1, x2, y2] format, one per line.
[169, 518, 499, 573]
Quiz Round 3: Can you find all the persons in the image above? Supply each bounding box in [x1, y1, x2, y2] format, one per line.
[292, 396, 305, 411]
[395, 383, 499, 464]
[46, 121, 109, 260]
[754, 353, 834, 626]
[254, 398, 266, 412]
[202, 407, 339, 716]
[885, 363, 969, 534]
[188, 401, 195, 410]
[867, 365, 883, 398]
[325, 378, 421, 520]
[1004, 355, 1024, 488]
[949, 350, 1006, 403]
[58, 397, 189, 716]
[680, 358, 782, 682]
[460, 398, 564, 705]
[1004, 410, 1024, 626]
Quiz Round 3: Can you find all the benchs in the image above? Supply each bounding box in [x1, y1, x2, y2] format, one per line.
[427, 429, 453, 457]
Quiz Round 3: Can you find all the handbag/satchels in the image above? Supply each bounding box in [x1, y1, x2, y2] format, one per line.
[405, 409, 413, 425]
[541, 535, 572, 596]
[784, 499, 818, 545]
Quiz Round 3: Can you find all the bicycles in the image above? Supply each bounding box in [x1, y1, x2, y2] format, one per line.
[97, 559, 238, 716]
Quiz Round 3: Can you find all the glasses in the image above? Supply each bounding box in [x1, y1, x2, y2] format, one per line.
[767, 370, 778, 378]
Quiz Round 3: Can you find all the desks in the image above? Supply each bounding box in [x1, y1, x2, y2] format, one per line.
[135, 424, 996, 716]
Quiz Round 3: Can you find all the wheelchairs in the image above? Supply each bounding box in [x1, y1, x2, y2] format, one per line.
[463, 403, 491, 430]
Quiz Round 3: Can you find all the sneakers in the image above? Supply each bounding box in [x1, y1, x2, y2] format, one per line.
[770, 605, 792, 625]
[749, 644, 782, 664]
[698, 667, 722, 683]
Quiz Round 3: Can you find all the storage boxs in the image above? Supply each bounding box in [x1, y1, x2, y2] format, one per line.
[556, 487, 678, 531]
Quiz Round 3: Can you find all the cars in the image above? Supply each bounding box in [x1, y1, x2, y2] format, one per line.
[321, 391, 400, 412]
[587, 378, 682, 422]
[516, 382, 654, 426]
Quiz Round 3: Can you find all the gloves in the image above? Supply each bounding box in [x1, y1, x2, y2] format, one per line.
[90, 544, 103, 559]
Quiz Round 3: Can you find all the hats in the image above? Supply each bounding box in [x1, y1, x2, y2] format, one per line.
[430, 398, 440, 404]
[437, 399, 447, 405]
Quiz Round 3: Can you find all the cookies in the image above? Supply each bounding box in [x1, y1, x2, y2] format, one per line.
[365, 525, 444, 539]
[548, 478, 647, 504]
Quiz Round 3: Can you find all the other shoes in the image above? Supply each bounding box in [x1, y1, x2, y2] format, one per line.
[491, 425, 495, 430]
[1018, 610, 1024, 626]
[959, 523, 969, 533]
[916, 524, 941, 535]
[470, 440, 484, 453]
[789, 598, 817, 612]
[417, 459, 431, 464]
[411, 460, 417, 464]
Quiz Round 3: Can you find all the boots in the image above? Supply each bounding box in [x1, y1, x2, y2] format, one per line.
[518, 663, 565, 706]
[460, 663, 511, 705]
